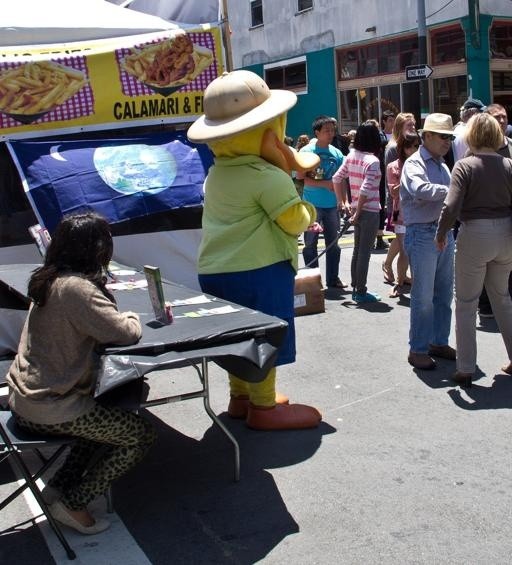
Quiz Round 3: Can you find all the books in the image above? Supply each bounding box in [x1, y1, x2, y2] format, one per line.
[143, 265, 169, 325]
[28, 223, 52, 257]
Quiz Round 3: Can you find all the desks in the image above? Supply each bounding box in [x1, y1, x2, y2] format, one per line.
[0, 242, 289, 514]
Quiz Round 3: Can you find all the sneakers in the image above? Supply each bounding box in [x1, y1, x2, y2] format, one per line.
[352, 289, 380, 304]
[381, 262, 411, 297]
[326, 280, 347, 288]
[408, 351, 437, 369]
[429, 343, 456, 360]
[376, 240, 391, 249]
[41, 484, 110, 535]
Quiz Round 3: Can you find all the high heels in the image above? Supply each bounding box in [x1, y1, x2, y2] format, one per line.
[502, 360, 512, 373]
[450, 371, 474, 388]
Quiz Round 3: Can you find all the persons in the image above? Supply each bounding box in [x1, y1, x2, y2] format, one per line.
[399, 112, 457, 371]
[5, 210, 158, 535]
[387, 127, 421, 297]
[297, 116, 348, 287]
[332, 123, 382, 302]
[435, 112, 511, 388]
[186, 70, 321, 429]
[285, 100, 511, 284]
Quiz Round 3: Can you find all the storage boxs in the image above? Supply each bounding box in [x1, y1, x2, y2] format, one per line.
[294, 266, 326, 320]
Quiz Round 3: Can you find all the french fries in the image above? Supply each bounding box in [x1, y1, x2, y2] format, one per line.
[0, 61, 86, 114]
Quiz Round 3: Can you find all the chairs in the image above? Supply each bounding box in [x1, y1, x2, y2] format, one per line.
[0, 410, 82, 559]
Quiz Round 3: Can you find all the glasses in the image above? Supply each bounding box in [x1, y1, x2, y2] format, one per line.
[437, 133, 456, 141]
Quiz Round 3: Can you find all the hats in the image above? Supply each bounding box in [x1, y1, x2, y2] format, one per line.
[418, 113, 456, 136]
[464, 99, 486, 110]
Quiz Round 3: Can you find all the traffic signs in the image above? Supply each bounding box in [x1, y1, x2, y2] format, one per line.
[404, 63, 435, 82]
